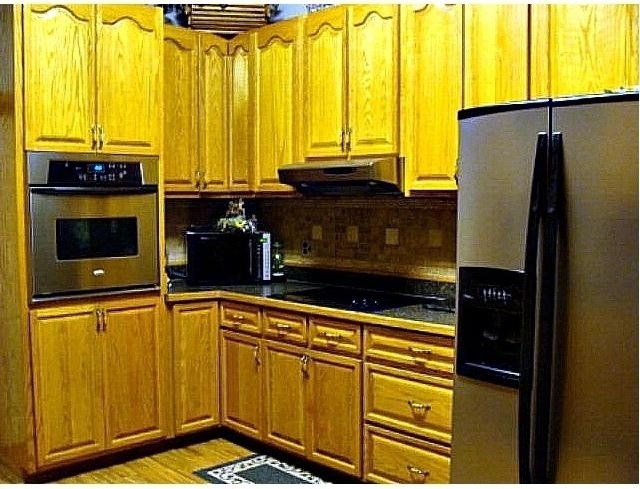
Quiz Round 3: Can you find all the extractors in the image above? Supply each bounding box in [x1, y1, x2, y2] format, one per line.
[276, 158, 405, 197]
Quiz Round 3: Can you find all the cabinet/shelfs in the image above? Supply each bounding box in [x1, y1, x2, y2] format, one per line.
[28, 301, 166, 464]
[163, 24, 233, 191]
[403, 3, 463, 193]
[464, 3, 530, 109]
[171, 301, 220, 437]
[529, 4, 640, 100]
[21, 2, 162, 154]
[221, 299, 263, 441]
[308, 5, 402, 160]
[307, 314, 363, 478]
[362, 324, 454, 485]
[262, 306, 307, 458]
[232, 16, 305, 193]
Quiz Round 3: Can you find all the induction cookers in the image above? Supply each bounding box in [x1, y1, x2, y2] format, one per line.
[270, 284, 434, 314]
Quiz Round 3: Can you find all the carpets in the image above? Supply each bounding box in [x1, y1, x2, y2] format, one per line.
[190, 454, 331, 484]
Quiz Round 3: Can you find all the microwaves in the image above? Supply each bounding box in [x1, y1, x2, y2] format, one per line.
[182, 229, 272, 286]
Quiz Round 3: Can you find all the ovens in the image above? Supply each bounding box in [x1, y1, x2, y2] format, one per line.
[25, 159, 160, 306]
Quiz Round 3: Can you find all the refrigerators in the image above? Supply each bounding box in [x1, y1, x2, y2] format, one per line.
[449, 93, 638, 484]
